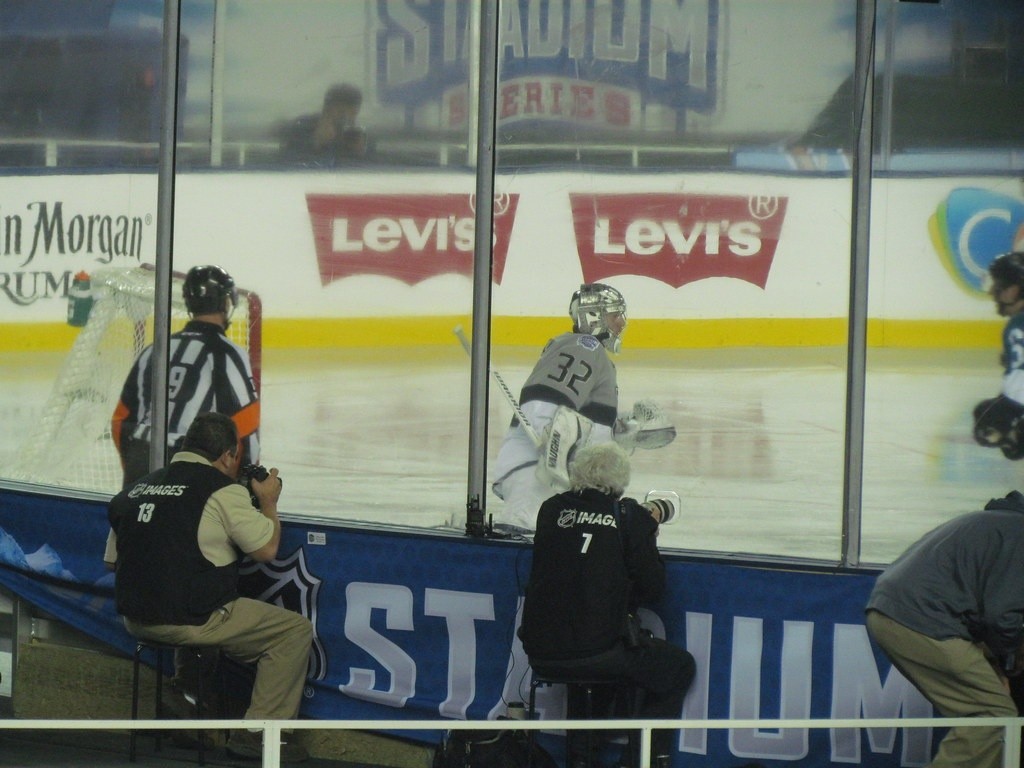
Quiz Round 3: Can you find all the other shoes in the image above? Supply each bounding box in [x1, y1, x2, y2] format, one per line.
[567, 759, 606, 768]
[621, 752, 662, 768]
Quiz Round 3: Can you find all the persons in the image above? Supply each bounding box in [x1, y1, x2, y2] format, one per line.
[863, 489, 1024, 768]
[102, 411, 314, 763]
[515, 441, 697, 768]
[972, 251, 1024, 462]
[491, 283, 677, 541]
[109, 264, 261, 490]
[276, 79, 371, 167]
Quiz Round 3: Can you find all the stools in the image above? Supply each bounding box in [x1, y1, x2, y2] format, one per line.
[128, 639, 229, 768]
[526, 676, 640, 768]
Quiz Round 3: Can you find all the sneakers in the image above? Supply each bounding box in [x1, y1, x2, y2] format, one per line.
[156, 683, 216, 750]
[225, 729, 309, 763]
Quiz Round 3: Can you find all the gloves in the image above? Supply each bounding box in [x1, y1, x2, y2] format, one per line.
[974, 393, 1024, 461]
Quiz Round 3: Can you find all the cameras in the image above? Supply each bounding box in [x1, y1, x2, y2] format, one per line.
[238, 464, 270, 508]
[641, 499, 675, 525]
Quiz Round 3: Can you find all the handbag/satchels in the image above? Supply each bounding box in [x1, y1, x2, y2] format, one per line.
[432, 716, 558, 768]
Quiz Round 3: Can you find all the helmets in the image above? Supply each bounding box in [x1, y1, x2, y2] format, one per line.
[991, 252, 1024, 300]
[183, 265, 239, 331]
[569, 284, 626, 353]
